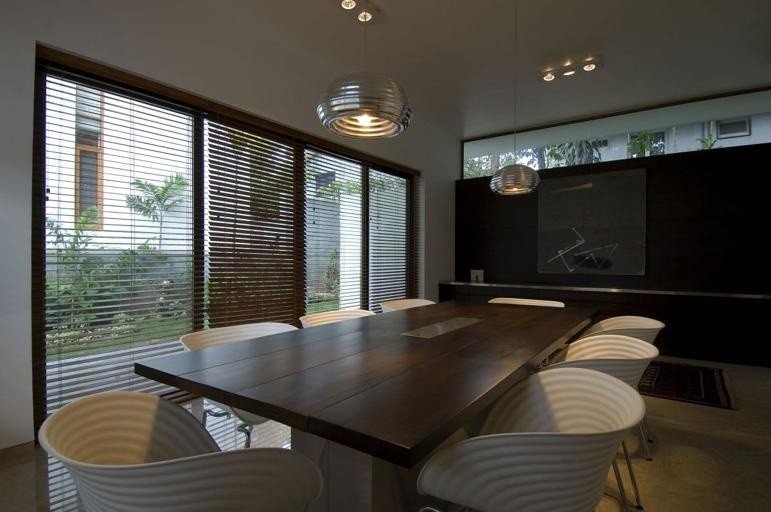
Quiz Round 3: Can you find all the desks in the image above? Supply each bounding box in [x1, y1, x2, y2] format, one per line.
[132, 301, 598, 509]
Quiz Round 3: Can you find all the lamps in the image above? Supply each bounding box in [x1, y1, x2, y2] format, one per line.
[316, 0, 413, 138]
[489, 0, 540, 197]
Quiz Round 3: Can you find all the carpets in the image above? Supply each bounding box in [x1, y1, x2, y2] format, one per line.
[638, 360, 737, 413]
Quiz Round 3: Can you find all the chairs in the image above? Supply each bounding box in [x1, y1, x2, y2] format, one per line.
[178, 322, 300, 448]
[35, 391, 325, 511]
[379, 298, 435, 312]
[489, 297, 566, 308]
[546, 335, 658, 466]
[417, 367, 644, 512]
[577, 314, 665, 352]
[299, 308, 376, 330]
